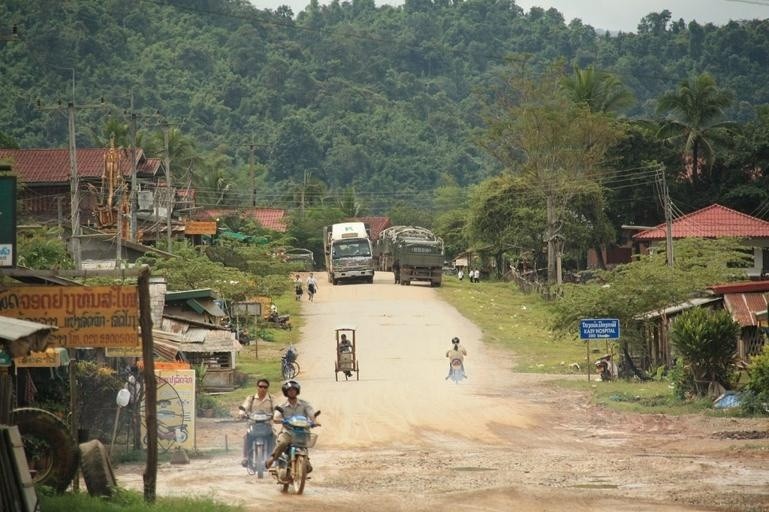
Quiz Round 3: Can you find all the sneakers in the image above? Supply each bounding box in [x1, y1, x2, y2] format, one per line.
[264, 455, 274, 469]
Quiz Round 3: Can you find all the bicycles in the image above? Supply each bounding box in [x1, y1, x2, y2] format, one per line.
[280, 346, 299, 380]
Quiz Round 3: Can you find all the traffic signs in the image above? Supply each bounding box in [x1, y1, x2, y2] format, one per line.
[579, 318, 620, 340]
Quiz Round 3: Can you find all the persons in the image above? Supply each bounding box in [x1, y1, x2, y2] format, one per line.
[338, 334, 354, 353]
[239, 380, 277, 466]
[294, 274, 304, 301]
[469, 268, 474, 283]
[445, 337, 467, 380]
[263, 378, 318, 474]
[306, 271, 319, 302]
[474, 267, 480, 283]
[392, 257, 402, 284]
[457, 269, 465, 281]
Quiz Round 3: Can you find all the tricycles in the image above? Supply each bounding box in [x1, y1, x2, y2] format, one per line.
[334, 329, 359, 382]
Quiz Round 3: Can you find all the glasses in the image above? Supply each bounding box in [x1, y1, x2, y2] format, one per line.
[258, 385, 268, 388]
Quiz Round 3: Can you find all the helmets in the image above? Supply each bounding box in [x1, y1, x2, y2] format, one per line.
[451, 337, 460, 344]
[281, 380, 300, 397]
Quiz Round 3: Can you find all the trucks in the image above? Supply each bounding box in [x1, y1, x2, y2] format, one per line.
[377, 225, 444, 287]
[322, 222, 374, 285]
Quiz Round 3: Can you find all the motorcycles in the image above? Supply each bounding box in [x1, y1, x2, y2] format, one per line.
[238, 405, 275, 479]
[594, 354, 620, 381]
[445, 354, 466, 385]
[269, 406, 321, 493]
